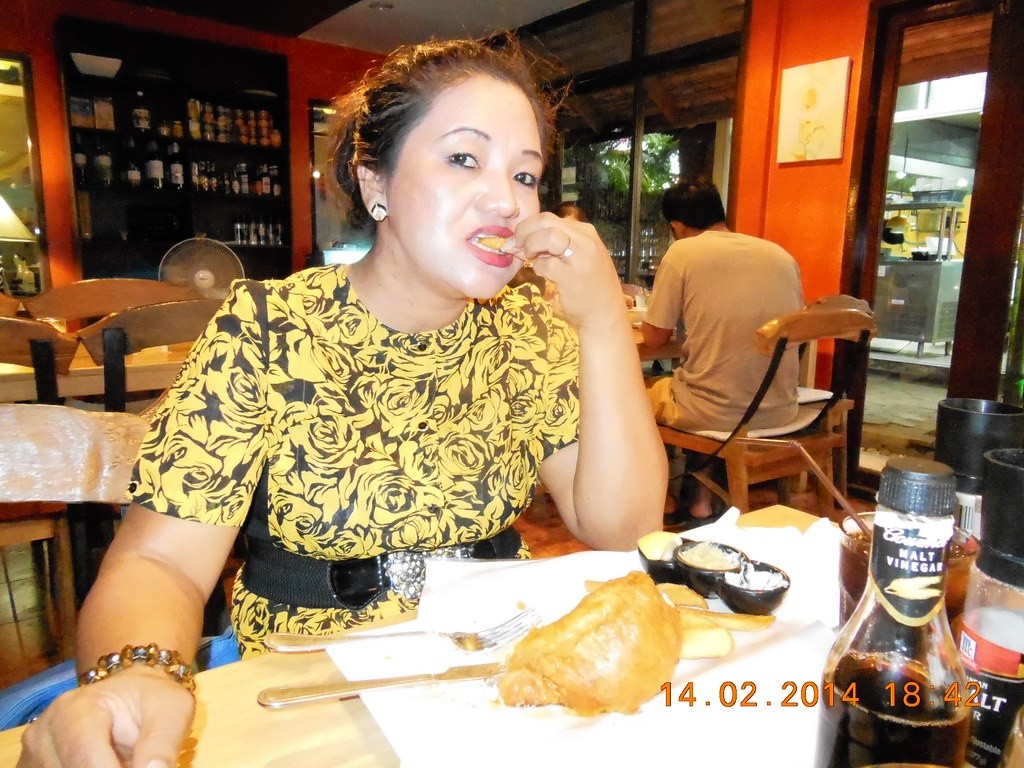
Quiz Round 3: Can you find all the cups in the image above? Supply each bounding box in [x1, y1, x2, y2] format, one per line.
[838, 511, 980, 630]
[634, 294, 649, 310]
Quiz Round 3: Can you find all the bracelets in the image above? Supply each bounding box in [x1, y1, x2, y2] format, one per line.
[77, 643, 197, 695]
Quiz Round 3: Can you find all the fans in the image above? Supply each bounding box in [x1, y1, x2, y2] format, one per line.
[158, 238, 245, 288]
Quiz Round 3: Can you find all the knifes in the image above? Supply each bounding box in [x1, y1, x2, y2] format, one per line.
[258, 663, 505, 708]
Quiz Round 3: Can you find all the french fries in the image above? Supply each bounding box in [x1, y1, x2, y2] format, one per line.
[477, 237, 509, 249]
[584, 580, 775, 660]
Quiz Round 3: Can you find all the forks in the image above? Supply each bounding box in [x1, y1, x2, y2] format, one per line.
[266, 608, 541, 652]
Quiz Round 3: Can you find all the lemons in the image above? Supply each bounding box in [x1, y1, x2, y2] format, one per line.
[636, 530, 682, 561]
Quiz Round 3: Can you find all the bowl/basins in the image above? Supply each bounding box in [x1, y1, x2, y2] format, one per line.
[719, 561, 789, 615]
[673, 542, 748, 599]
[637, 537, 693, 585]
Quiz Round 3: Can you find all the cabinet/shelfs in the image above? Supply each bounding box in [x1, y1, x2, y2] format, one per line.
[54, 13, 292, 280]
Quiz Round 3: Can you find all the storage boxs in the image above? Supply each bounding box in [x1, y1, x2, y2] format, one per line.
[912, 189, 968, 201]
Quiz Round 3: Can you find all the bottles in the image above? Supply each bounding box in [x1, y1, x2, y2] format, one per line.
[71, 132, 89, 184]
[159, 98, 281, 147]
[93, 134, 113, 185]
[169, 141, 183, 189]
[933, 398, 1024, 538]
[815, 456, 971, 767]
[132, 91, 150, 130]
[120, 136, 141, 185]
[143, 140, 164, 188]
[234, 222, 282, 246]
[192, 161, 281, 197]
[956, 447, 1024, 768]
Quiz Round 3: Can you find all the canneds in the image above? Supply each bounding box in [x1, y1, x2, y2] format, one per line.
[160, 98, 280, 148]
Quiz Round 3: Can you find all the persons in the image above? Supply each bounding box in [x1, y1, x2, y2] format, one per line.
[641, 180, 803, 528]
[18, 25, 668, 768]
[552, 204, 634, 309]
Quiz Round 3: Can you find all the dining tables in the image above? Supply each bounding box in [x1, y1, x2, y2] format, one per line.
[534, 327, 826, 496]
[0, 504, 841, 768]
[0, 343, 199, 403]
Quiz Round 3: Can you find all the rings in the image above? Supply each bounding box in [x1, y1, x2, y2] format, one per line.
[556, 236, 573, 260]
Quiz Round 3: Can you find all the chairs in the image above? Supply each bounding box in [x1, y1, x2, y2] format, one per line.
[0, 279, 879, 661]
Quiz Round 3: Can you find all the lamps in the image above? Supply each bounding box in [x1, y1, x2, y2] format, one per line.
[0, 194, 37, 292]
[885, 136, 910, 227]
